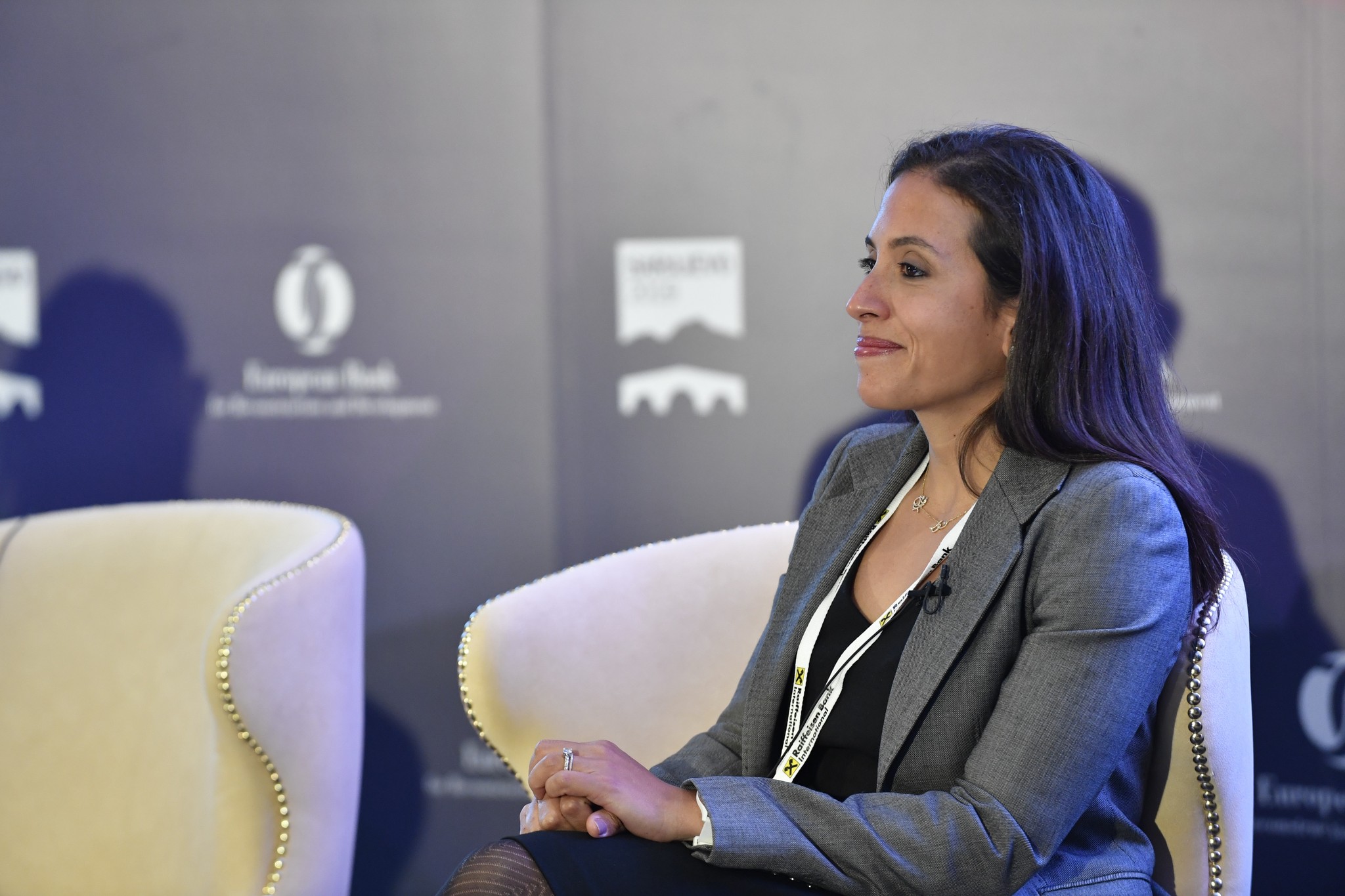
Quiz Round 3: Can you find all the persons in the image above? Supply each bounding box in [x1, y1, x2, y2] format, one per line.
[441, 126, 1223, 896]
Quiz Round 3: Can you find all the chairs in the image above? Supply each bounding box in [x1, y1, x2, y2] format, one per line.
[0, 500, 366, 896]
[458, 521, 1257, 896]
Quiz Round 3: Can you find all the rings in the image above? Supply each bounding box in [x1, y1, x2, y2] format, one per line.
[564, 749, 574, 771]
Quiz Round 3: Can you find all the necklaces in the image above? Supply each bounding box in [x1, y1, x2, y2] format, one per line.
[911, 465, 969, 532]
